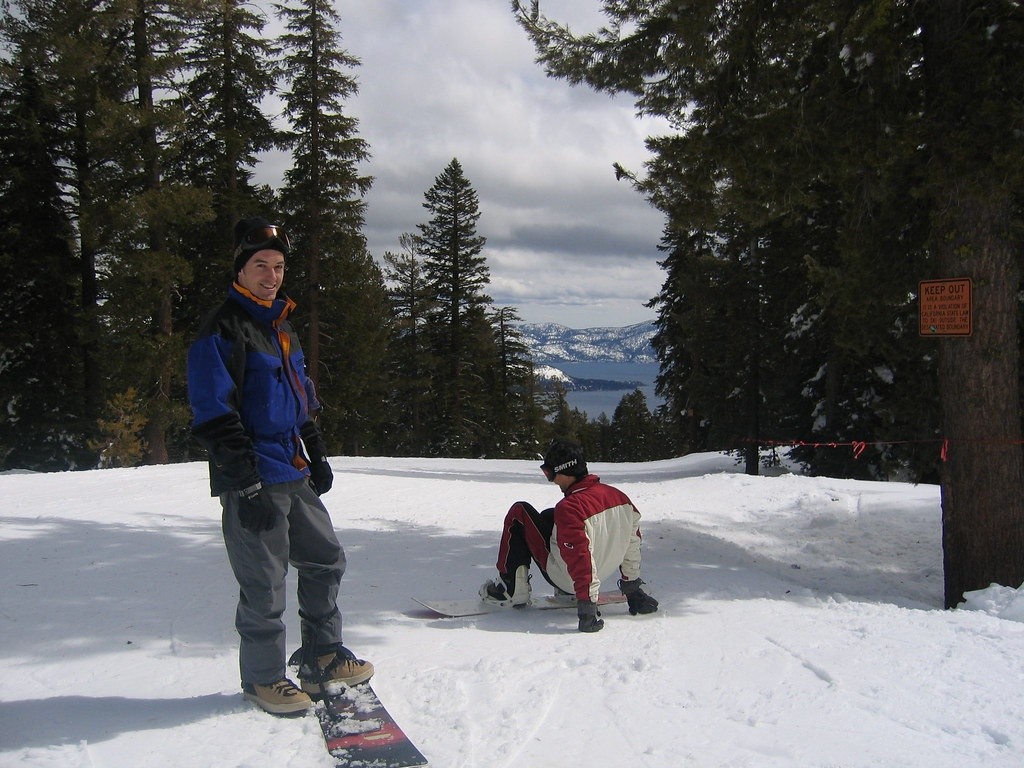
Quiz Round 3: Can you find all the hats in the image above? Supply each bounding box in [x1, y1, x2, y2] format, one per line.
[233, 217, 288, 273]
[543, 440, 588, 477]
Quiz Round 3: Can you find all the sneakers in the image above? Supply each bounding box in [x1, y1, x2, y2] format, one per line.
[297, 642, 374, 694]
[241, 676, 311, 714]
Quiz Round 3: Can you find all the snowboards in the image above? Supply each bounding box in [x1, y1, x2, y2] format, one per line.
[287, 644, 430, 768]
[412, 588, 626, 617]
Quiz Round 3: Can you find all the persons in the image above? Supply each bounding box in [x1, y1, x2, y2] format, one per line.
[189, 220, 375, 713]
[478, 442, 660, 632]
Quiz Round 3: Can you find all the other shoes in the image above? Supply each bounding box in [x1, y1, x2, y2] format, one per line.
[478, 573, 516, 601]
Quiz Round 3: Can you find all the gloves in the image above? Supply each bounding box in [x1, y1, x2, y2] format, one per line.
[235, 473, 274, 535]
[311, 456, 332, 494]
[576, 599, 604, 632]
[617, 577, 658, 615]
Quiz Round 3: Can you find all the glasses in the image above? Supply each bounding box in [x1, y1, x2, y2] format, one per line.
[540, 454, 584, 482]
[233, 225, 293, 262]
